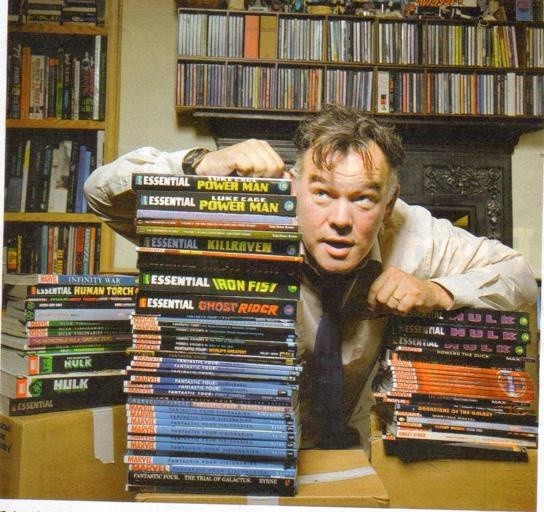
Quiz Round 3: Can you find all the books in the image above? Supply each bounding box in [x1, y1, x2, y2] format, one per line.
[6, 0, 107, 275]
[3, 271, 140, 417]
[374, 300, 538, 458]
[177, 14, 544, 120]
[122, 169, 304, 496]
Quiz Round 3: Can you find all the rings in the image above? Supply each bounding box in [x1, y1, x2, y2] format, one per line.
[392, 294, 402, 303]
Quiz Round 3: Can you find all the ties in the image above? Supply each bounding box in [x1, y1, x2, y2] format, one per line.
[302, 260, 364, 450]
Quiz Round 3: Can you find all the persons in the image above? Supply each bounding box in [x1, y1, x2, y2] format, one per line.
[80, 102, 538, 451]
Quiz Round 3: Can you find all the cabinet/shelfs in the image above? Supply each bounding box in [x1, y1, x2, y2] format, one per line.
[175, 7, 544, 123]
[3, 0, 124, 275]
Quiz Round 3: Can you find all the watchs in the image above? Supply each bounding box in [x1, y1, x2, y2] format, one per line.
[181, 146, 212, 176]
